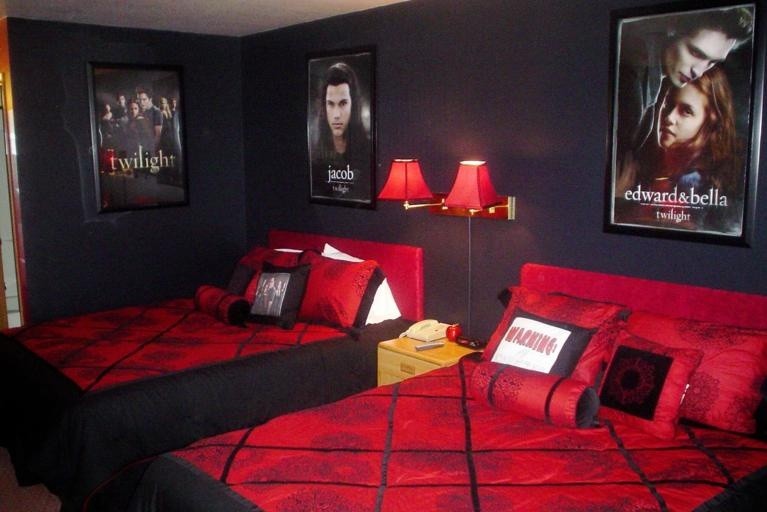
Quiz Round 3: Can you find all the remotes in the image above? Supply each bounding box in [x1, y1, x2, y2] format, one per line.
[416, 341, 445, 351]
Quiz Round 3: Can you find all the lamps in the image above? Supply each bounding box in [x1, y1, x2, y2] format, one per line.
[377, 154, 510, 219]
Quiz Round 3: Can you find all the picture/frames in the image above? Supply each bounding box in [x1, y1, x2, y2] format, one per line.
[83, 56, 192, 215]
[304, 42, 378, 209]
[602, 3, 765, 249]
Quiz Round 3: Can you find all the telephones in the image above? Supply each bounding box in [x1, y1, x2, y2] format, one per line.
[407, 319, 450, 342]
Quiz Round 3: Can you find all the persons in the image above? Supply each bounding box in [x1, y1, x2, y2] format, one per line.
[252, 272, 289, 319]
[312, 63, 371, 201]
[639, 66, 744, 237]
[614, 5, 756, 194]
[92, 82, 183, 188]
